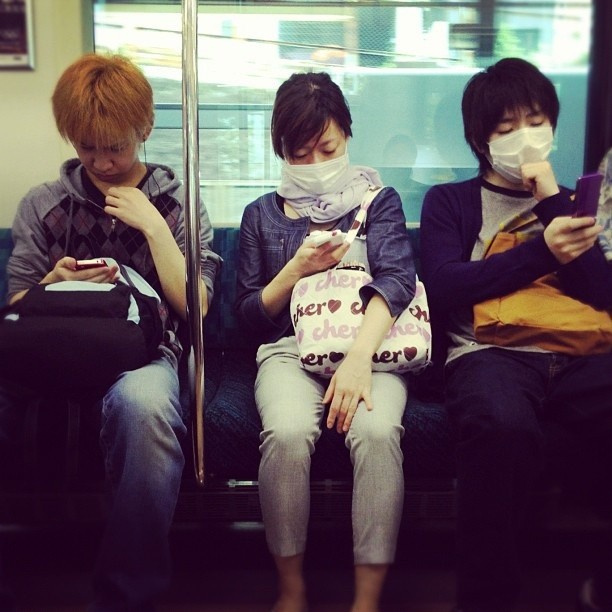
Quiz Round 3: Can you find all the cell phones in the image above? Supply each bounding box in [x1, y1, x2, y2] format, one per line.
[76, 258, 109, 272]
[571, 172, 604, 229]
[312, 228, 344, 253]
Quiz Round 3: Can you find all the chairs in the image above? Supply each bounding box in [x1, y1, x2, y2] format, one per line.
[201, 227, 427, 537]
[0, 225, 201, 538]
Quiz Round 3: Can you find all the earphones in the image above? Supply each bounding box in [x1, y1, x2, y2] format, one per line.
[141, 127, 148, 140]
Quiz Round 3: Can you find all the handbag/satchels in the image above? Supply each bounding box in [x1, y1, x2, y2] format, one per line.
[285, 184, 435, 375]
[472, 192, 612, 366]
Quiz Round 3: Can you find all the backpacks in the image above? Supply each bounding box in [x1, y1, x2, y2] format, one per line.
[0, 258, 169, 398]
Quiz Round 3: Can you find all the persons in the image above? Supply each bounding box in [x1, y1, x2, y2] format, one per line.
[231, 71, 417, 612]
[3, 55, 225, 611]
[419, 56, 612, 611]
